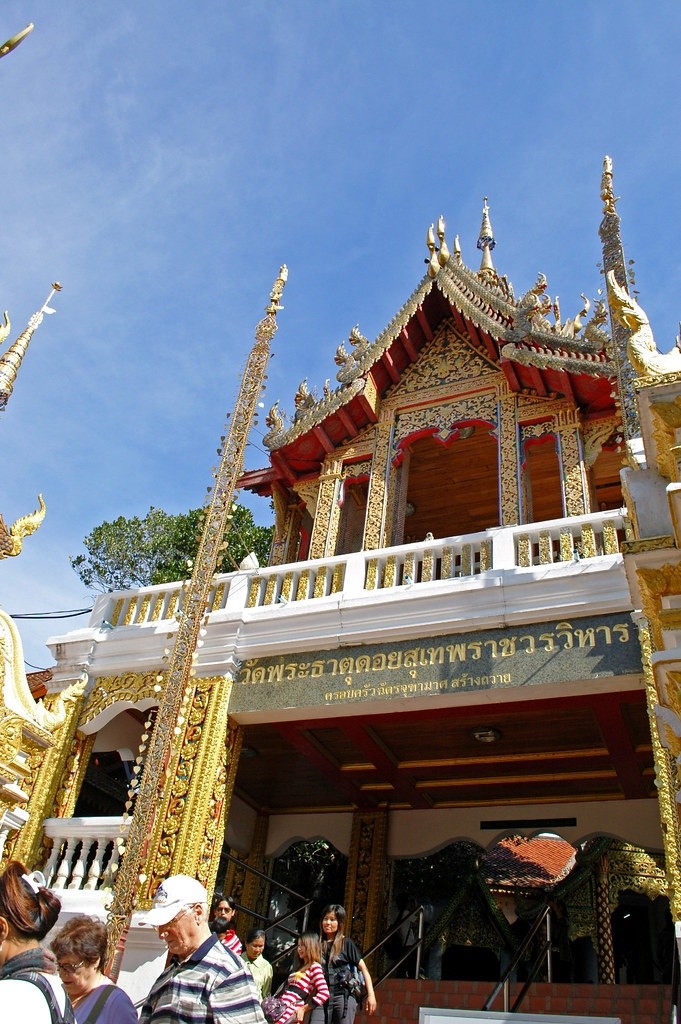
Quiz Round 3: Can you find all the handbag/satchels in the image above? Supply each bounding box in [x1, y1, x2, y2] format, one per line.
[337, 964, 366, 996]
[261, 993, 287, 1022]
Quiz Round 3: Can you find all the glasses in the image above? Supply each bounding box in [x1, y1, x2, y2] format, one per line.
[153, 903, 197, 932]
[216, 907, 231, 914]
[57, 960, 84, 973]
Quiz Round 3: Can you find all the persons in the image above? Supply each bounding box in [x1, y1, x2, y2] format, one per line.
[0, 860, 377, 1024]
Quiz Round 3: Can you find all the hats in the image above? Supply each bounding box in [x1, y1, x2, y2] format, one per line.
[138, 875, 207, 926]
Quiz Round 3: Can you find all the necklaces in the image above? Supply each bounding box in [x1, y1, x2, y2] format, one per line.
[71, 974, 102, 1005]
